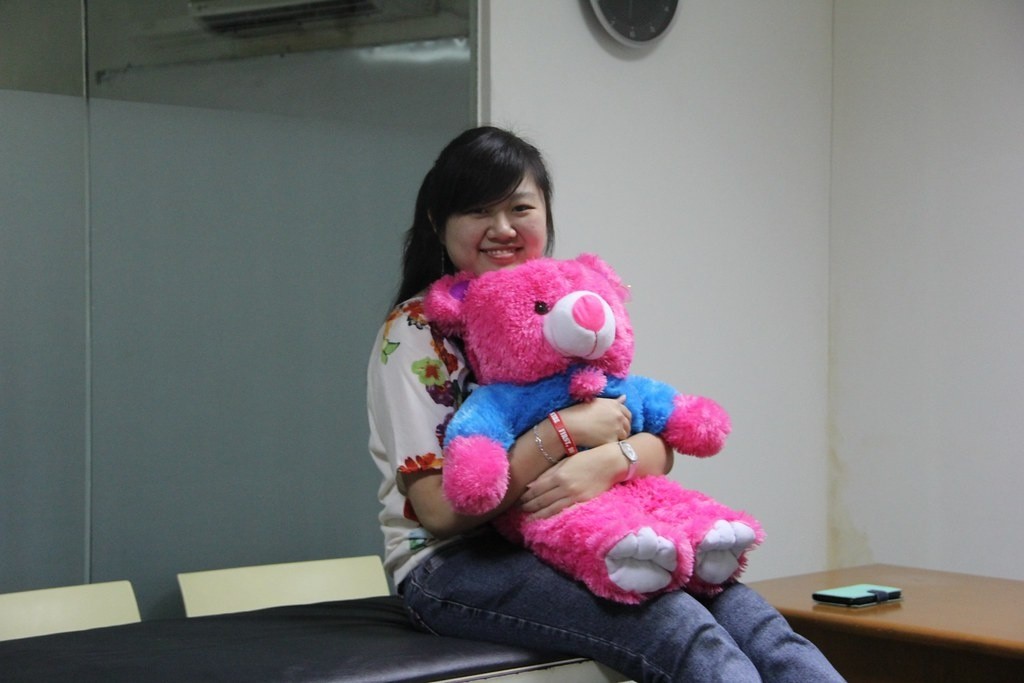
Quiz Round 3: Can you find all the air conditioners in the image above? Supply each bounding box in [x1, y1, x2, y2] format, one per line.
[188, 0, 440, 41]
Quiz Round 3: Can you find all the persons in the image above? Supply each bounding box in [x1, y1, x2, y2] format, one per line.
[366, 125, 855, 683]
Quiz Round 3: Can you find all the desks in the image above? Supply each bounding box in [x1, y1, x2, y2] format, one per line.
[0, 595, 630, 683]
[742, 564, 1024, 683]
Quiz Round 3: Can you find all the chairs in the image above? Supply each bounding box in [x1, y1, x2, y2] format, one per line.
[0, 579, 142, 641]
[177, 555, 391, 617]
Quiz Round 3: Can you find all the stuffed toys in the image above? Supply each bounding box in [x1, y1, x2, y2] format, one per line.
[427, 254, 765, 601]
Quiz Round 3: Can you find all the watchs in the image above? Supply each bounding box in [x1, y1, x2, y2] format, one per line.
[618, 440, 638, 483]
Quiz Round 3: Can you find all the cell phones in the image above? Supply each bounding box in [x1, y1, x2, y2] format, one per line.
[813, 584, 903, 608]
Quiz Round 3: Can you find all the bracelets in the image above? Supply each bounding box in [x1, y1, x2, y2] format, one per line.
[535, 428, 559, 466]
[549, 412, 577, 455]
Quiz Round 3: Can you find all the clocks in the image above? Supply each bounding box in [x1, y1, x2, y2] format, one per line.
[590, 0, 684, 48]
